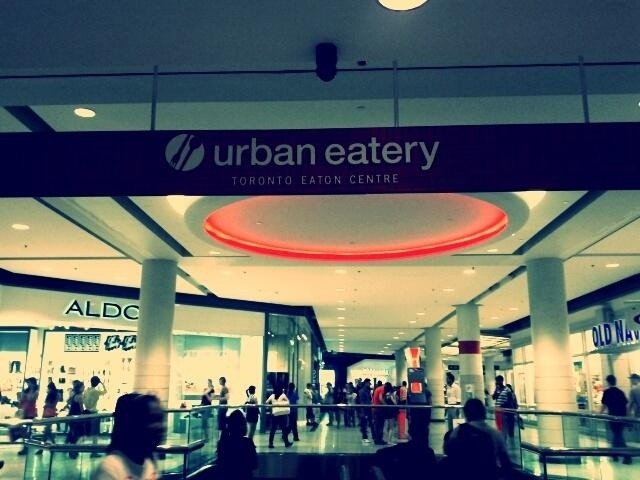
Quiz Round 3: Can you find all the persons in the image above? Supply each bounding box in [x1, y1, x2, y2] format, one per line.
[87, 393, 167, 480]
[442, 427, 454, 458]
[266, 382, 301, 449]
[484, 373, 519, 445]
[443, 373, 462, 430]
[600, 374, 633, 465]
[626, 374, 640, 431]
[243, 385, 260, 441]
[209, 376, 230, 439]
[303, 378, 431, 447]
[218, 408, 258, 479]
[0, 375, 108, 472]
[444, 396, 542, 480]
[376, 419, 437, 479]
[200, 378, 216, 442]
